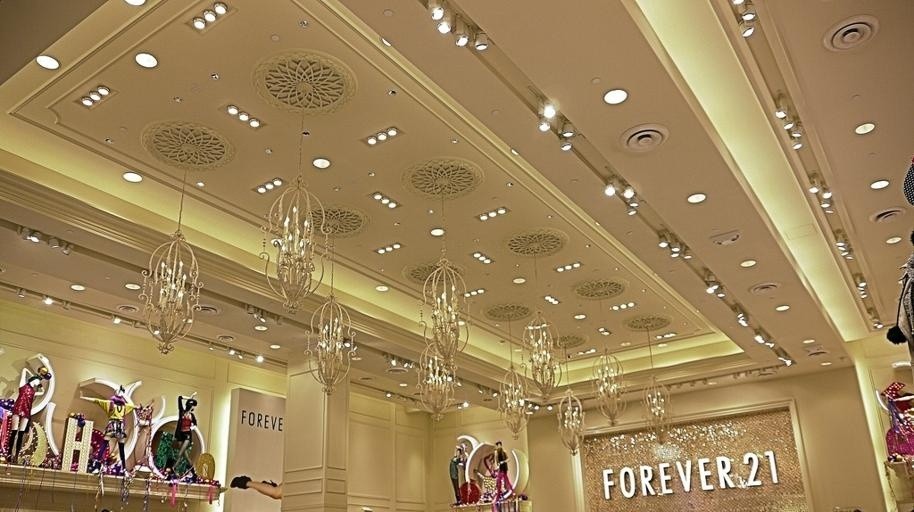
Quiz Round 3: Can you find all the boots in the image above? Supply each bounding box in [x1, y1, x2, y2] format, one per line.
[261, 478, 278, 488]
[232, 475, 251, 491]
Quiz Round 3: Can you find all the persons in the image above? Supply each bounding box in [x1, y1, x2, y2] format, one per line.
[81, 388, 142, 477]
[493, 441, 515, 501]
[230, 474, 283, 499]
[164, 390, 201, 482]
[6, 367, 45, 463]
[450, 444, 468, 504]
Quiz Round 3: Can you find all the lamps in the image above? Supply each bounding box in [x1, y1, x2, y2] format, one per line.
[0, 0, 882, 456]
